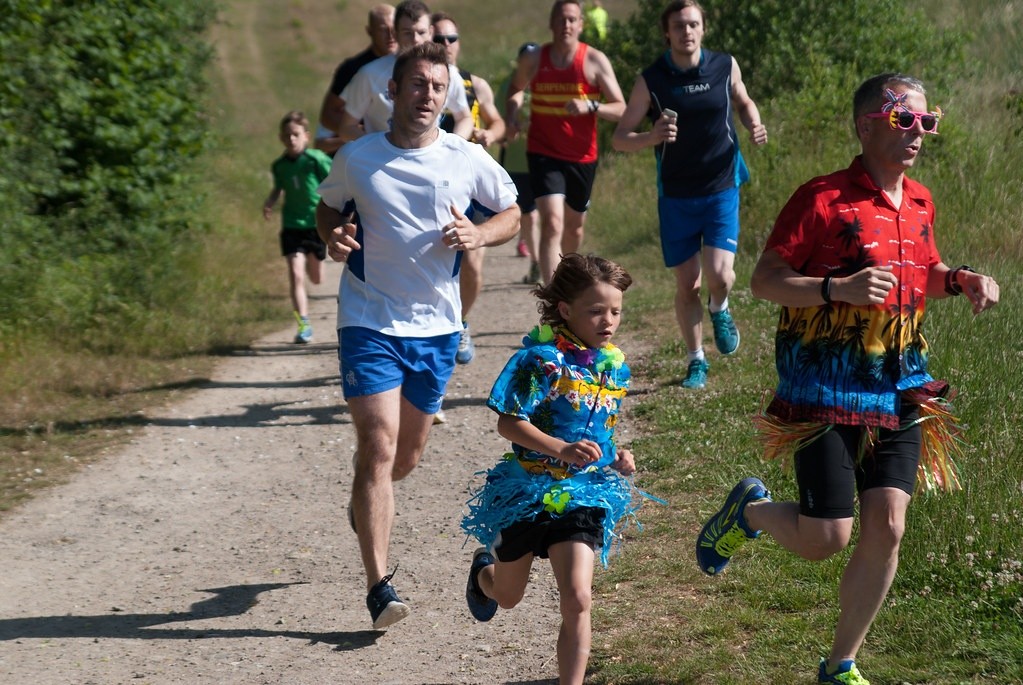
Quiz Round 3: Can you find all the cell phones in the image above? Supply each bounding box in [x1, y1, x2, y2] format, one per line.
[663, 108, 678, 127]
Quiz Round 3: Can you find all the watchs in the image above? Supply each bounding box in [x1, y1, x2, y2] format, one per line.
[591, 99, 599, 113]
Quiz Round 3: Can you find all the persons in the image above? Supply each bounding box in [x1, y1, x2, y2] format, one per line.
[612, 0, 769, 390]
[313, 0, 540, 365]
[505, 0, 626, 366]
[460, 253, 667, 685]
[263, 111, 334, 343]
[315, 42, 520, 629]
[580, 0, 608, 40]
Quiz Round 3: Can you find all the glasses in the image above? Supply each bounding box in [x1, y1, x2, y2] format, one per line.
[865, 111, 938, 134]
[433, 34, 460, 44]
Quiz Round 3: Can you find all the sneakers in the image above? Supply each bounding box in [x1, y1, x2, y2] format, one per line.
[346, 450, 359, 535]
[466, 547, 498, 622]
[683, 357, 710, 389]
[518, 238, 530, 258]
[818, 660, 869, 685]
[366, 563, 410, 630]
[709, 293, 740, 355]
[528, 262, 541, 285]
[696, 478, 771, 576]
[294, 311, 312, 343]
[455, 319, 474, 364]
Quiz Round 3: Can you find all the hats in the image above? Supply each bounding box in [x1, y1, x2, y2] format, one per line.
[518, 42, 539, 57]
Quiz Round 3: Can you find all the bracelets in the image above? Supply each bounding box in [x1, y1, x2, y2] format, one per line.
[584, 99, 594, 114]
[696, 73, 1000, 685]
[821, 276, 834, 305]
[952, 264, 976, 293]
[944, 268, 961, 296]
